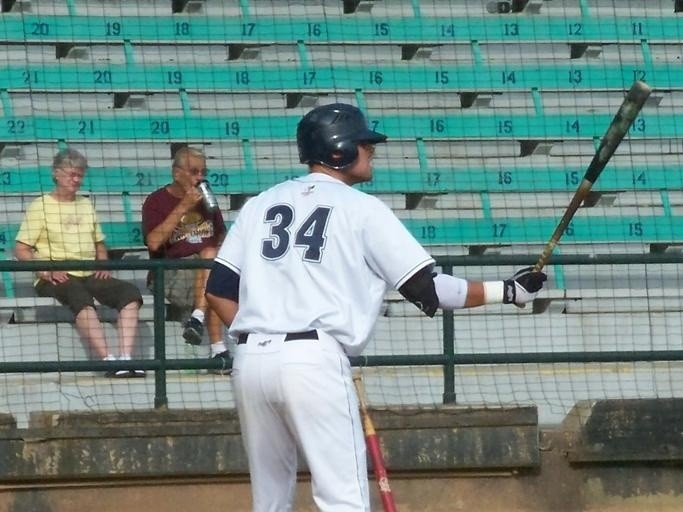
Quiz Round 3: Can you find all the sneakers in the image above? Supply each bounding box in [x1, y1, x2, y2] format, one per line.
[208, 351, 232, 375]
[183, 317, 204, 345]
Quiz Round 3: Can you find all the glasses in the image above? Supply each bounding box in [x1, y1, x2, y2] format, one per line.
[176, 164, 208, 176]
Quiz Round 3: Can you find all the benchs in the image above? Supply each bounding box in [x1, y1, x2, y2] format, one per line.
[0, 0, 683, 323]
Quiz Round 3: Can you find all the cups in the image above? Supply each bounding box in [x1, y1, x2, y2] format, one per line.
[195, 179, 218, 213]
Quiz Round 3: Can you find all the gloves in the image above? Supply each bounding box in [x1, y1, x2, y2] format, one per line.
[504, 267, 546, 304]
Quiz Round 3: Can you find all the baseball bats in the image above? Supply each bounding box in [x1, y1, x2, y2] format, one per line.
[354, 376, 396, 511]
[515, 81, 652, 309]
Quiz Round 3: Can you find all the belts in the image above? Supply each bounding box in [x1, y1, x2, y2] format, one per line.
[237, 330, 316, 341]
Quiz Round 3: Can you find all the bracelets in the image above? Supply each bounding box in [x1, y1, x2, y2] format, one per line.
[483, 280, 504, 304]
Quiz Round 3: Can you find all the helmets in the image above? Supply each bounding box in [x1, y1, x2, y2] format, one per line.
[297, 103, 386, 170]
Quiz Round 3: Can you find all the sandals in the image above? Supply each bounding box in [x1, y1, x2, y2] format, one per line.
[105, 368, 145, 378]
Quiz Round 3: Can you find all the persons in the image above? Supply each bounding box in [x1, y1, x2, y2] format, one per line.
[142, 147, 232, 374]
[15, 149, 145, 378]
[205, 103, 548, 512]
